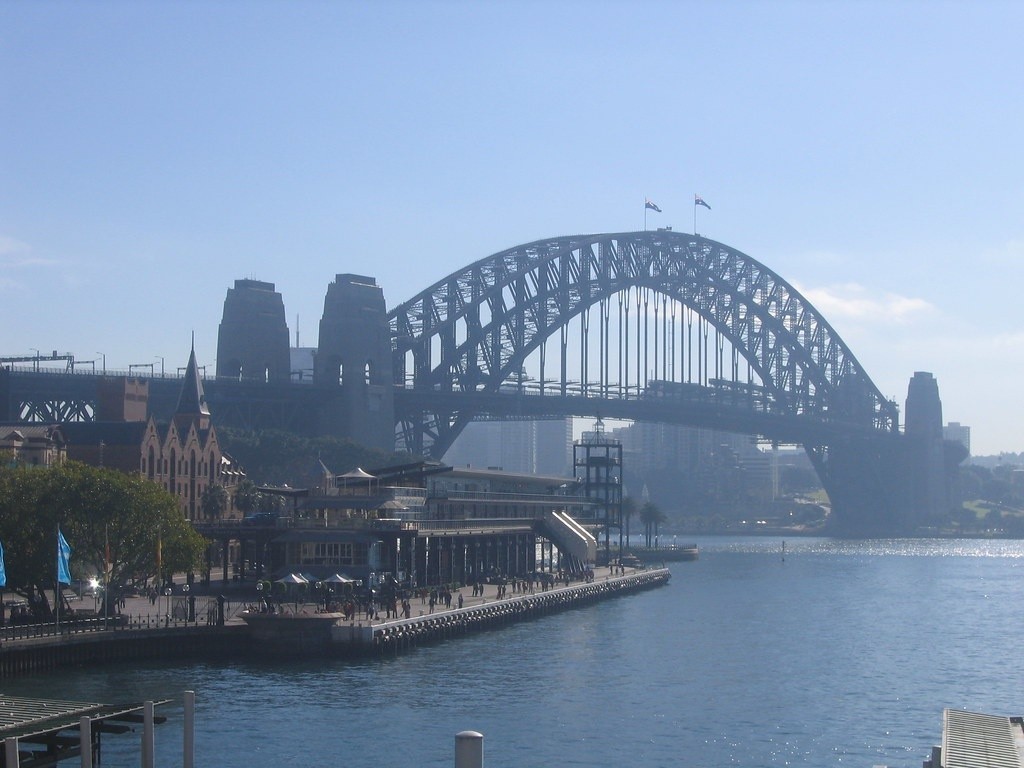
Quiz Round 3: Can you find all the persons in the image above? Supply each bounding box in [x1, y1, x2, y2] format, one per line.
[321, 564, 625, 619]
[119, 584, 159, 608]
[260, 599, 275, 614]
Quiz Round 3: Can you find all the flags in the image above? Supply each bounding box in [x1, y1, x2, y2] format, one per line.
[695, 195, 711, 210]
[645, 199, 662, 212]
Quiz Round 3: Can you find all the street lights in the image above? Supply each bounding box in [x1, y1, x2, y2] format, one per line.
[315, 581, 322, 613]
[183, 584, 190, 626]
[93, 591, 100, 615]
[164, 588, 173, 626]
[256, 583, 263, 614]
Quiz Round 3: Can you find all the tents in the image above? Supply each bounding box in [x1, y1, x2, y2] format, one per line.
[336, 467, 409, 518]
[270, 571, 363, 601]
